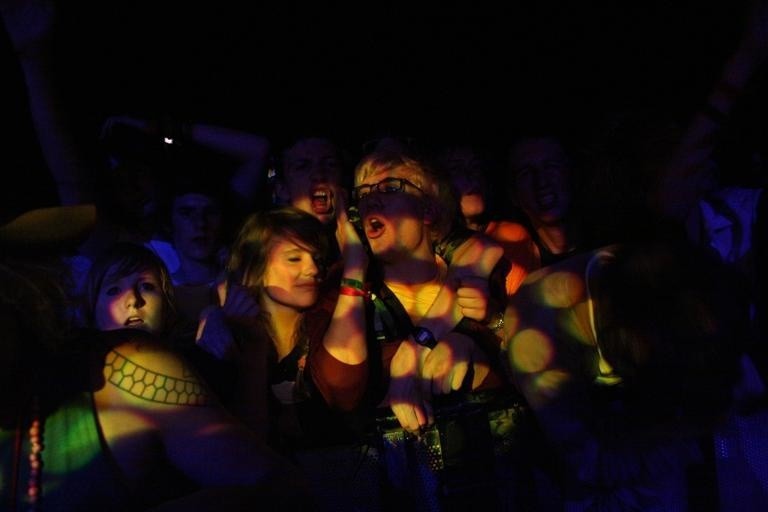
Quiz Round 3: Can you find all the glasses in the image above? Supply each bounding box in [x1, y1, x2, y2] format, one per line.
[351, 178, 425, 201]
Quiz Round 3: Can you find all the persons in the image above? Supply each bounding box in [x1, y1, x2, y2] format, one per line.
[0, 122, 768, 512]
